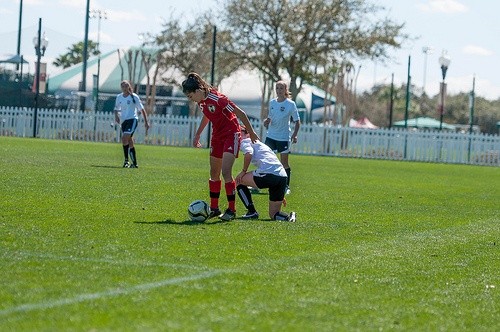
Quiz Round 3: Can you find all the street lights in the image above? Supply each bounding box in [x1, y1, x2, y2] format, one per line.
[32, 31, 49, 137]
[438, 49, 452, 132]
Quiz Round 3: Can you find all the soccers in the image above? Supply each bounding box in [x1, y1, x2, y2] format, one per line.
[188, 200, 211, 222]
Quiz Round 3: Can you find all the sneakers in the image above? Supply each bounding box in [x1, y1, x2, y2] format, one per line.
[288, 212, 297, 223]
[248, 187, 261, 193]
[285, 185, 290, 194]
[242, 211, 258, 219]
[209, 208, 220, 218]
[221, 208, 236, 221]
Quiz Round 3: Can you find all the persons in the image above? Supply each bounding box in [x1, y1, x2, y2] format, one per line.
[248, 80, 301, 194]
[114, 80, 150, 168]
[182, 72, 260, 221]
[235, 125, 296, 222]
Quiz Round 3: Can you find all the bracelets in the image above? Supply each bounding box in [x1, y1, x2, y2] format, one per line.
[243, 169, 247, 172]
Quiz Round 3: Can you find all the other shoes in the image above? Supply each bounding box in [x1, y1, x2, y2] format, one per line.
[130, 164, 138, 168]
[123, 161, 129, 167]
